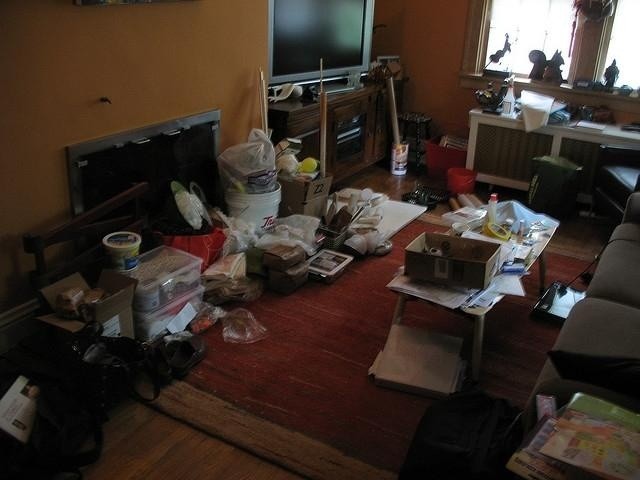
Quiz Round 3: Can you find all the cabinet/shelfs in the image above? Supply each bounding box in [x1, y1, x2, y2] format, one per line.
[330, 94, 368, 169]
[267, 102, 332, 185]
[374, 90, 395, 160]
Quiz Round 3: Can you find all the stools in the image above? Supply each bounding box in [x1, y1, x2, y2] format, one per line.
[398, 110, 432, 170]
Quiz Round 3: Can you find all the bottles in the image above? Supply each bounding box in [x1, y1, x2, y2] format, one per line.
[487, 194, 498, 223]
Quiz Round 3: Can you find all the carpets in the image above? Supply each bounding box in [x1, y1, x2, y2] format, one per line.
[137, 218, 598, 475]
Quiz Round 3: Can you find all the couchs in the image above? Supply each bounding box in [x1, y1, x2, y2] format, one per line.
[512, 191, 640, 480]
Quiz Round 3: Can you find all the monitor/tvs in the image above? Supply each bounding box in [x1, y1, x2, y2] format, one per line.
[267, 1, 376, 96]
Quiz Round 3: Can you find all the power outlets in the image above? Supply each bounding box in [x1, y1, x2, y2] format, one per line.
[376, 54, 402, 65]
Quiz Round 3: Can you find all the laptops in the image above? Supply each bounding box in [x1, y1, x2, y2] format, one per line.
[530, 281, 586, 322]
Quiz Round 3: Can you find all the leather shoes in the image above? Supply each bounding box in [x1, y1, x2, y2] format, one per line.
[169, 333, 208, 378]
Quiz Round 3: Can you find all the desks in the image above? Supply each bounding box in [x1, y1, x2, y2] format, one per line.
[465, 104, 640, 209]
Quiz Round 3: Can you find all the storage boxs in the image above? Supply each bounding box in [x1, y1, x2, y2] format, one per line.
[404, 230, 502, 290]
[268, 258, 309, 297]
[424, 132, 468, 179]
[131, 285, 206, 342]
[290, 178, 331, 218]
[261, 240, 305, 272]
[116, 245, 203, 310]
[315, 228, 349, 251]
[32, 269, 136, 341]
[368, 323, 466, 401]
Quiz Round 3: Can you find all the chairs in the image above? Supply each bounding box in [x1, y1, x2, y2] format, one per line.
[593, 140, 640, 216]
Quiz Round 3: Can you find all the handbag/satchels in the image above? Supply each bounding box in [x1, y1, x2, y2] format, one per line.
[60, 319, 161, 413]
